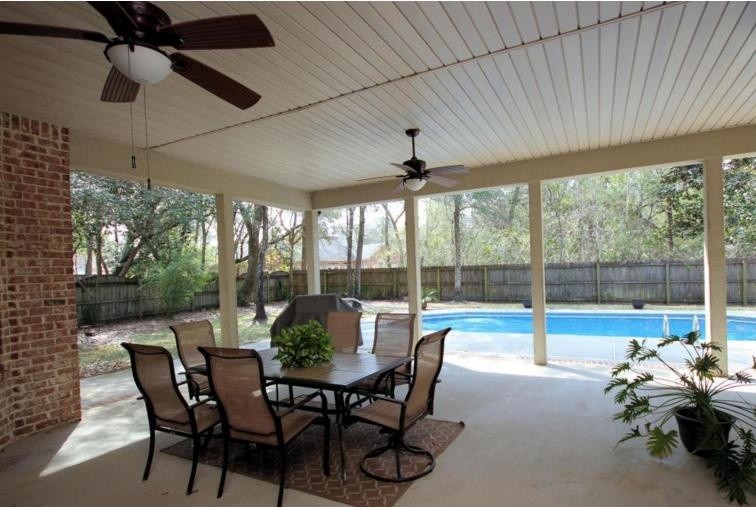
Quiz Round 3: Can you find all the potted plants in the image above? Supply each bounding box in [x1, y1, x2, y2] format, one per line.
[421, 287, 438, 309]
[603, 329, 756, 507]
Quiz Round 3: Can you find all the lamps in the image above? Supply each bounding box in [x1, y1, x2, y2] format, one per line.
[404, 178, 428, 191]
[103, 39, 174, 85]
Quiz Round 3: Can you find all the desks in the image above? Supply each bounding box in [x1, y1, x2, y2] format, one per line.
[198, 345, 412, 477]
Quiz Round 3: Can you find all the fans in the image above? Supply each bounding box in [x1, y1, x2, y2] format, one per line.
[0, 1, 275, 110]
[355, 127, 466, 194]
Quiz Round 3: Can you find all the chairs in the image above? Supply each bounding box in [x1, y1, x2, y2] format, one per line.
[197, 346, 332, 507]
[325, 311, 364, 353]
[353, 313, 417, 407]
[120, 342, 251, 496]
[344, 326, 451, 483]
[169, 319, 218, 402]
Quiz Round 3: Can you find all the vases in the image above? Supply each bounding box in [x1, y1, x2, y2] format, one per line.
[522, 299, 532, 308]
[631, 297, 645, 309]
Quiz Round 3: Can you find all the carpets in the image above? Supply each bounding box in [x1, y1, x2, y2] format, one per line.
[161, 396, 465, 507]
